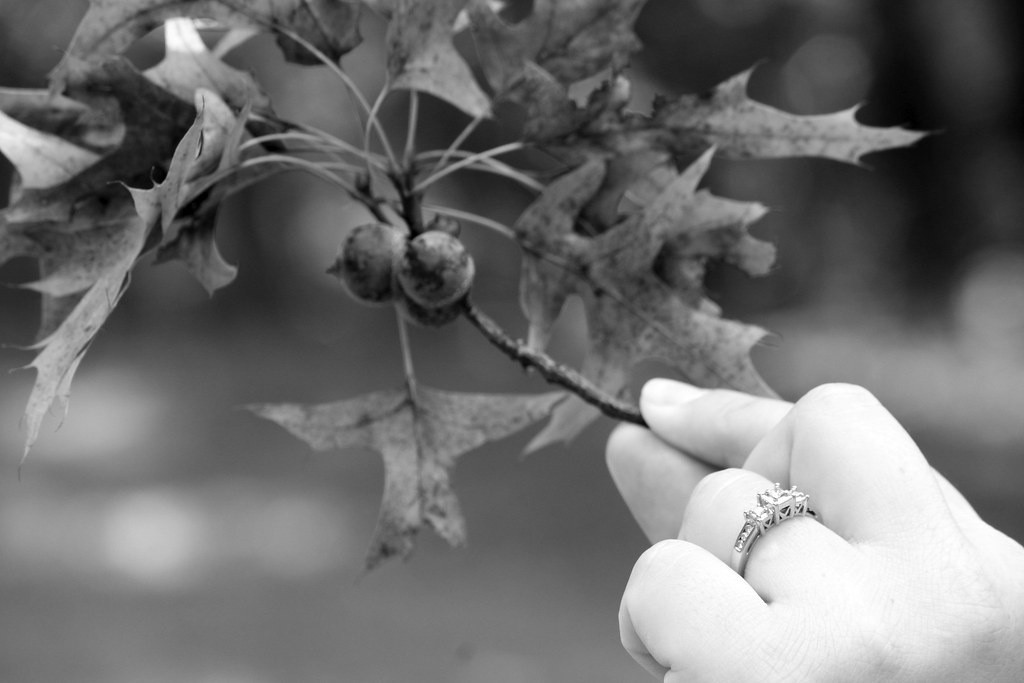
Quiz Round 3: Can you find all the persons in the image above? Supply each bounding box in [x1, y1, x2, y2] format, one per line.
[606, 376, 1024, 683]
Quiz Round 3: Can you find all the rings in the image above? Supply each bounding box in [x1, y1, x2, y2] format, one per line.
[731, 483, 819, 579]
